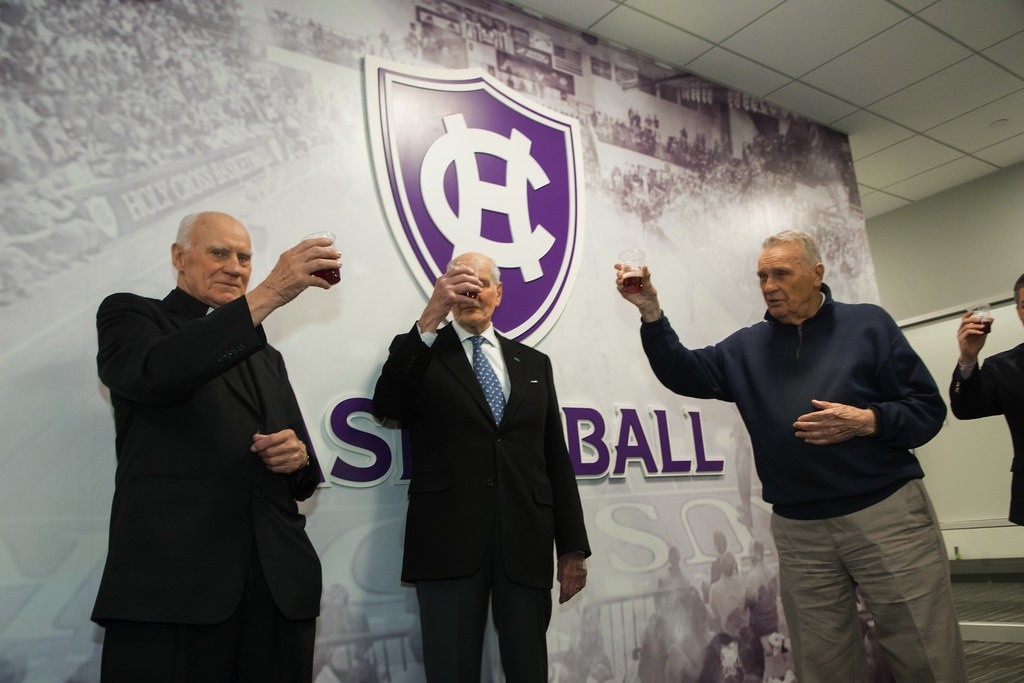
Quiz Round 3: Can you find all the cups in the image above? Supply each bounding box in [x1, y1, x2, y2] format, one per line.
[452, 261, 479, 300]
[618, 249, 647, 292]
[303, 231, 341, 284]
[969, 302, 990, 335]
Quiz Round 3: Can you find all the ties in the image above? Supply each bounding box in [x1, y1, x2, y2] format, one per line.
[466, 336, 507, 428]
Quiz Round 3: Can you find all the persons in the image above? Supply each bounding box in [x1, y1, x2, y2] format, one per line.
[1, 1, 881, 318]
[368, 249, 596, 683]
[556, 416, 797, 683]
[88, 206, 343, 683]
[948, 268, 1024, 526]
[613, 226, 978, 683]
